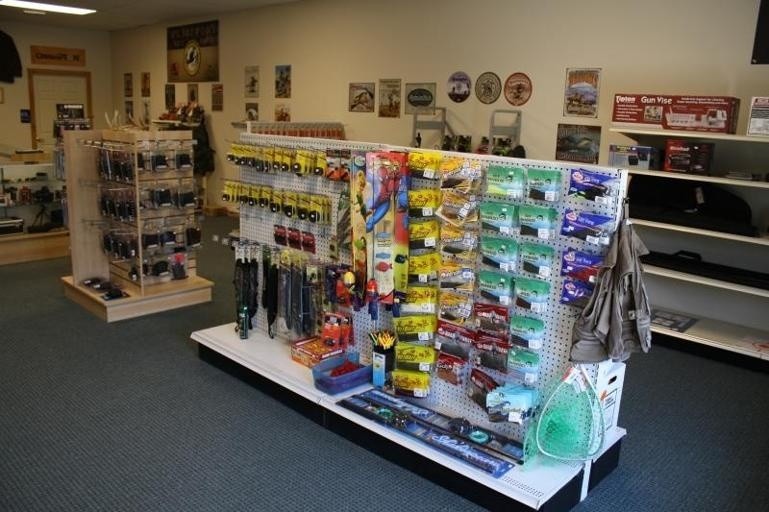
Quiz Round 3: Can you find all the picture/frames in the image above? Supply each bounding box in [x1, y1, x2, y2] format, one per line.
[563, 68, 602, 119]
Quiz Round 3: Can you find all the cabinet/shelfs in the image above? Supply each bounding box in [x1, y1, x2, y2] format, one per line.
[190, 132, 629, 512]
[59, 123, 215, 324]
[412, 106, 522, 160]
[0, 151, 72, 266]
[607, 127, 769, 362]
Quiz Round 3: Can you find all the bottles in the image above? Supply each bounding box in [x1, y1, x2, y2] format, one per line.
[239, 305, 250, 339]
